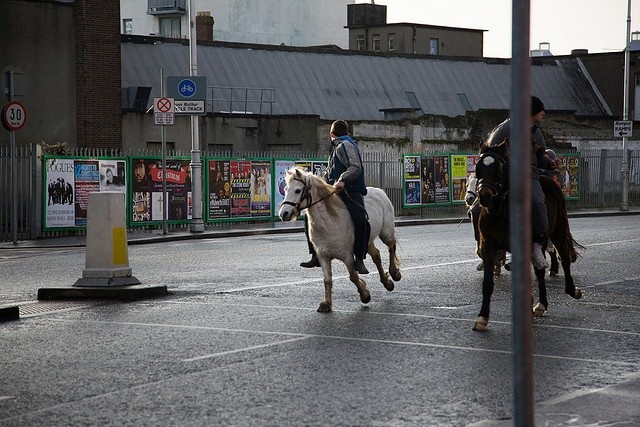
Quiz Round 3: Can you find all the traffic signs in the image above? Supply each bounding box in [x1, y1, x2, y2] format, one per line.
[613, 121, 632, 137]
[4, 101, 27, 129]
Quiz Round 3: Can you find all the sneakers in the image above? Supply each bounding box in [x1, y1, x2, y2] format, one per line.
[300, 254, 320, 268]
[353, 260, 370, 274]
[532, 242, 549, 270]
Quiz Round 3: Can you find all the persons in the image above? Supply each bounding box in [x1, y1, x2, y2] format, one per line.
[47, 177, 73, 206]
[252, 164, 269, 194]
[299, 119, 372, 273]
[106, 168, 122, 185]
[422, 167, 434, 203]
[476, 91, 562, 272]
[460, 181, 466, 199]
[540, 148, 561, 188]
[218, 189, 225, 199]
[436, 173, 449, 189]
[133, 159, 151, 186]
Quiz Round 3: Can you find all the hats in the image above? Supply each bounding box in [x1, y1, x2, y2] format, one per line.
[531, 95, 545, 117]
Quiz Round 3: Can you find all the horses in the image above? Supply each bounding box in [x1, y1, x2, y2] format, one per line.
[472, 137, 587, 332]
[278, 166, 401, 312]
[464, 173, 507, 271]
